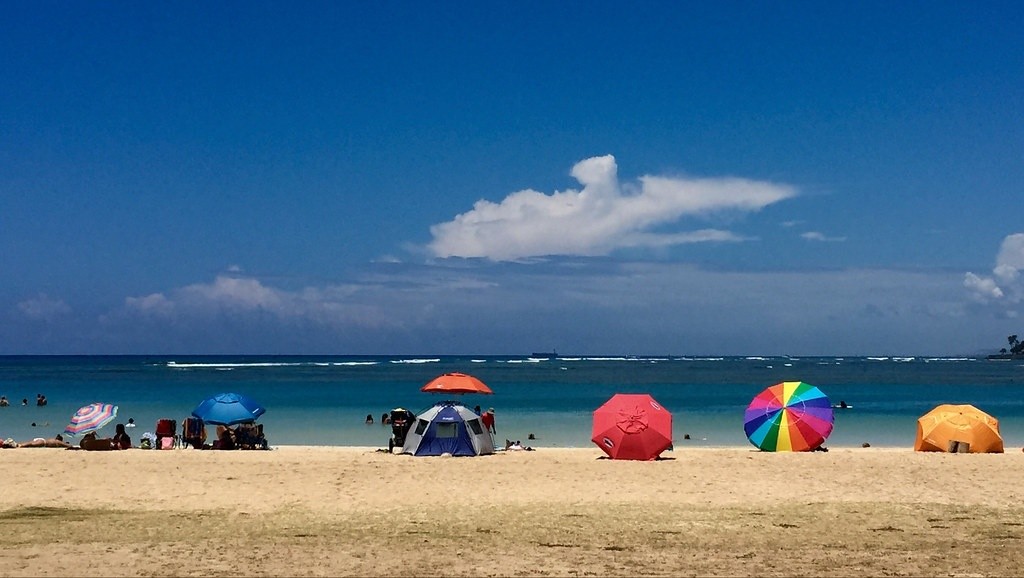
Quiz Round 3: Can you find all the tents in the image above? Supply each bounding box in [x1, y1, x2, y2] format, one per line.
[402, 402, 494, 455]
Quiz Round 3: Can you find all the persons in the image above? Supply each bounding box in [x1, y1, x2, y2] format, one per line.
[37, 394, 47, 405]
[591, 394, 672, 461]
[481, 408, 496, 435]
[474, 406, 481, 415]
[528, 434, 535, 439]
[21, 399, 28, 405]
[113, 424, 131, 446]
[125, 418, 136, 428]
[55, 433, 63, 441]
[217, 425, 269, 450]
[365, 414, 373, 423]
[685, 434, 691, 440]
[502, 438, 535, 450]
[0, 396, 8, 406]
[840, 401, 847, 408]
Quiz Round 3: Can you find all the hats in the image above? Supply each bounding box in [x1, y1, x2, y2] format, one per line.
[487, 408, 495, 414]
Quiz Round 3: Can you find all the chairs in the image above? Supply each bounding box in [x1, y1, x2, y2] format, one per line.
[154, 418, 268, 450]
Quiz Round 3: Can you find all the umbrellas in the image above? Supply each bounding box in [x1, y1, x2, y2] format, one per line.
[913, 403, 1004, 454]
[64, 403, 118, 437]
[192, 392, 267, 426]
[420, 372, 494, 394]
[743, 382, 835, 452]
